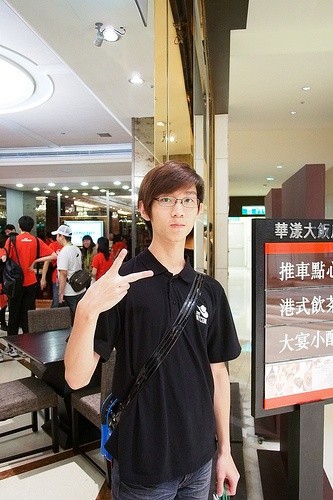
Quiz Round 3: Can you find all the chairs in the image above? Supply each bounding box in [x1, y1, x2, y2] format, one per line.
[71, 349, 113, 490]
[0, 377, 59, 453]
[28, 307, 72, 377]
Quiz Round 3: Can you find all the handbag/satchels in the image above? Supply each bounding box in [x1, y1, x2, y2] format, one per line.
[99, 390, 123, 463]
[67, 269, 91, 293]
[2, 235, 24, 299]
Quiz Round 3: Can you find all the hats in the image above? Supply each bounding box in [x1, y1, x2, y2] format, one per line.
[50, 224, 72, 238]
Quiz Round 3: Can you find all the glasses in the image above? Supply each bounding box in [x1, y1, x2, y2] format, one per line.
[153, 195, 201, 209]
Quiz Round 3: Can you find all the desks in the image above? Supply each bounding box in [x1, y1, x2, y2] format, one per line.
[4, 329, 103, 450]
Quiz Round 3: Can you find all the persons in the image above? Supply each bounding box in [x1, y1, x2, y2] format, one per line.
[81, 235, 97, 289]
[50, 225, 86, 327]
[64, 161, 240, 500]
[40, 240, 64, 308]
[0, 224, 18, 331]
[184, 227, 193, 269]
[108, 231, 149, 257]
[91, 237, 114, 281]
[37, 231, 55, 247]
[1, 216, 57, 357]
